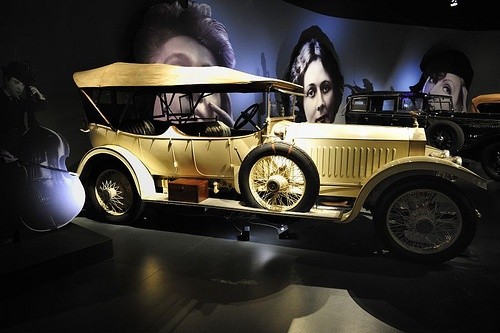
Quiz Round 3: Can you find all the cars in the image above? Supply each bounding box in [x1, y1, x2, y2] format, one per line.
[342, 91, 500, 181]
[73, 62, 489, 265]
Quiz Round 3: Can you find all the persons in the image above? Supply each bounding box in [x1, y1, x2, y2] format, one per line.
[410, 43, 474, 112]
[279, 25, 344, 123]
[0, 62, 49, 245]
[119, 0, 234, 128]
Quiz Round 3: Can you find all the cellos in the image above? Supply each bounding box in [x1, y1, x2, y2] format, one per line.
[18, 58, 87, 232]
